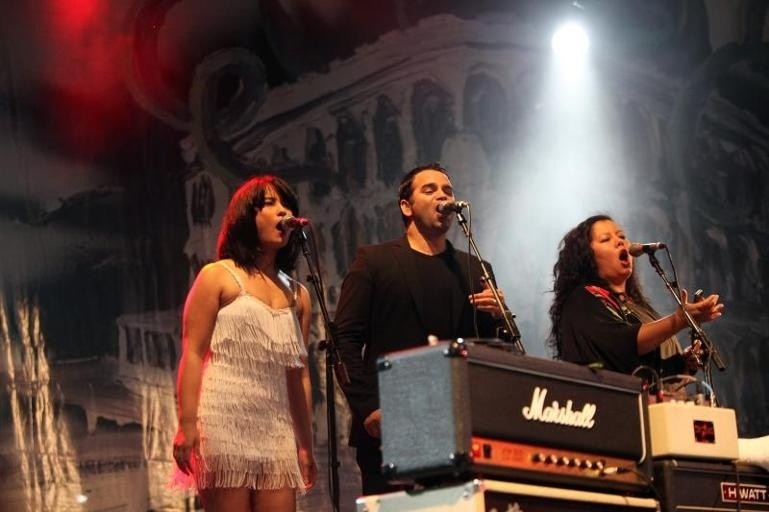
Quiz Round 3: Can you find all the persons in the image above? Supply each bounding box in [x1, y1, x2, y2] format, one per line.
[545, 215, 725, 396]
[171, 175, 321, 512]
[327, 162, 514, 496]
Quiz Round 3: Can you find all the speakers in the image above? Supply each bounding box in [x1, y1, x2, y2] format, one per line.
[652, 459, 769, 512]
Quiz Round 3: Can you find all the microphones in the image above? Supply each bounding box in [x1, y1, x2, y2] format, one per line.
[280, 214, 311, 232]
[438, 200, 469, 214]
[629, 242, 666, 258]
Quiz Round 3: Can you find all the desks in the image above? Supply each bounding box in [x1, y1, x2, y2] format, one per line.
[356, 480, 661, 512]
